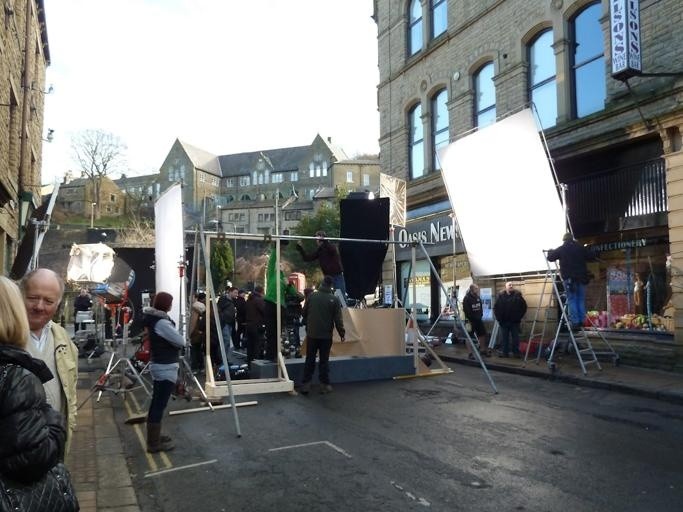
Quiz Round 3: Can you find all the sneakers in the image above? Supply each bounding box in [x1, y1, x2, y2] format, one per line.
[321, 384, 332, 395]
[300, 383, 310, 394]
[571, 325, 581, 331]
[580, 322, 586, 327]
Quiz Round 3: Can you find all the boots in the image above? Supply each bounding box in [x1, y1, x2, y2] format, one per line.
[147, 424, 175, 453]
[160, 435, 172, 442]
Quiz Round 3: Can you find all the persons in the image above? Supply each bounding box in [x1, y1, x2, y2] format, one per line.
[546, 232, 596, 331]
[186, 275, 318, 374]
[295, 230, 348, 307]
[16, 266, 84, 462]
[493, 281, 528, 359]
[462, 283, 491, 360]
[71, 288, 94, 332]
[300, 276, 347, 395]
[0, 274, 70, 485]
[140, 292, 195, 455]
[632, 272, 644, 307]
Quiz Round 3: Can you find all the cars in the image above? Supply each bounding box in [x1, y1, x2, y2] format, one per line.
[383, 303, 429, 322]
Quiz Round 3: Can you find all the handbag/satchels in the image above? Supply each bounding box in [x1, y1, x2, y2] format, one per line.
[0, 461, 79, 511]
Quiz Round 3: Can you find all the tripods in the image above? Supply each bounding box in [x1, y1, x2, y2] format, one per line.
[139, 270, 217, 415]
[77, 306, 157, 410]
[89, 312, 115, 358]
[89, 308, 154, 402]
[420, 219, 480, 347]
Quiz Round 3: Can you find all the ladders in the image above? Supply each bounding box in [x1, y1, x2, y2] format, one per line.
[522, 250, 603, 376]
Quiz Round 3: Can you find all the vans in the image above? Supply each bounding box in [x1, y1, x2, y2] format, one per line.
[360, 288, 381, 306]
